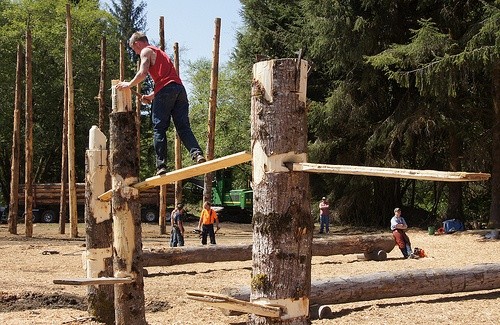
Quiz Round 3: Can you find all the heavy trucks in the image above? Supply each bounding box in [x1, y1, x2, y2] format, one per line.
[0, 201, 175, 223]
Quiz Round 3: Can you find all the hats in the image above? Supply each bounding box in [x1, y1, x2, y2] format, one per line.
[394, 208, 401, 212]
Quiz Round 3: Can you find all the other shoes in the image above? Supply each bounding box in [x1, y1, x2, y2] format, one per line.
[196, 155, 207, 164]
[405, 253, 419, 259]
[319, 231, 323, 234]
[156, 166, 167, 175]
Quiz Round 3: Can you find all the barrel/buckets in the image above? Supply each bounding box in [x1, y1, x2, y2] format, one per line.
[427, 227, 434, 234]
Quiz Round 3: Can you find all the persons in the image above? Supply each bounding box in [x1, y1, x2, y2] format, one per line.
[169, 201, 220, 246]
[318, 196, 330, 234]
[115, 32, 207, 176]
[390, 208, 419, 259]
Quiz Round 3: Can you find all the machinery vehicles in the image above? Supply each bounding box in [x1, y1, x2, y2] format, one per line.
[181, 166, 253, 224]
